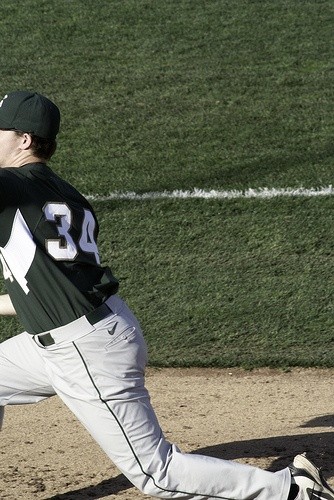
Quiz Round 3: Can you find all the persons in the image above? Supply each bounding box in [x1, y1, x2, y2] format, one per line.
[0, 91, 334, 500]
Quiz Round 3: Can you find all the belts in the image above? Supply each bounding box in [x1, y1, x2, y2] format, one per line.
[32, 302, 113, 349]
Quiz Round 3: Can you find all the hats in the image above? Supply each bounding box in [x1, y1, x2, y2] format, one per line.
[0, 90, 60, 139]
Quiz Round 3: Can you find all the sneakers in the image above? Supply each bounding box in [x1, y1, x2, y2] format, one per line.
[286, 451, 334, 500]
